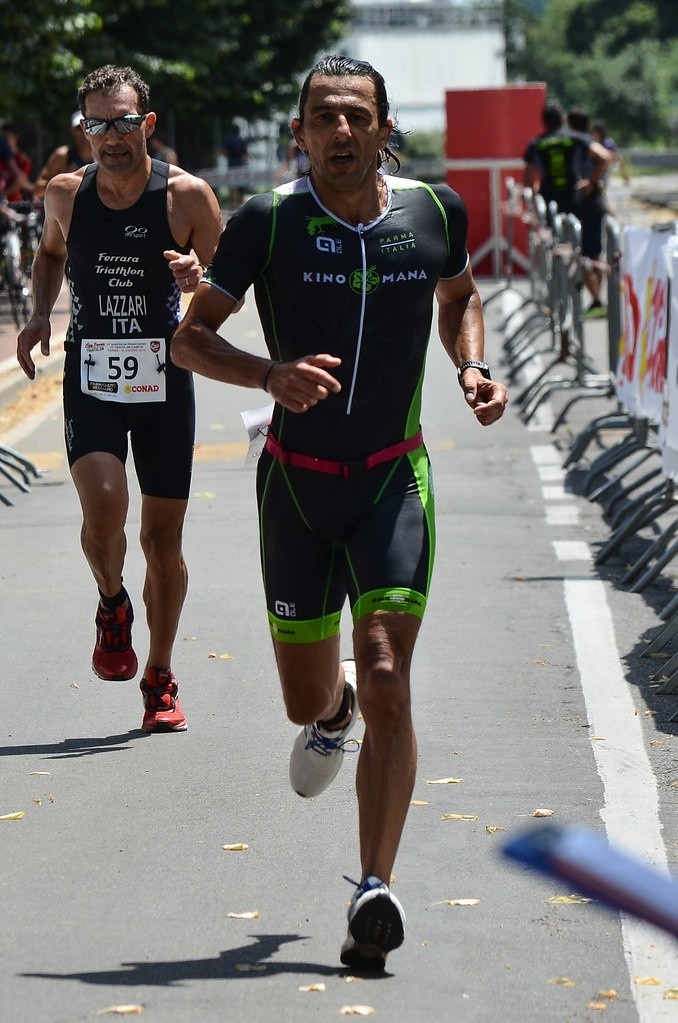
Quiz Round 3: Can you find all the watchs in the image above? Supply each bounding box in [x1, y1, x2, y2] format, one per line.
[457, 361, 491, 385]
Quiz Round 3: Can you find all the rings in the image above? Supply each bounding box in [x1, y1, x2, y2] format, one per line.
[185, 278, 189, 286]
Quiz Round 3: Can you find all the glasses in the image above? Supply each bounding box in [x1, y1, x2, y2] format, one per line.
[81, 114, 145, 136]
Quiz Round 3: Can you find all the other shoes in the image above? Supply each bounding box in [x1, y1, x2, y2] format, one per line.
[585, 305, 608, 318]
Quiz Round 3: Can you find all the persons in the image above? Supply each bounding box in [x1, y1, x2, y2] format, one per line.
[169, 57, 508, 975]
[521, 99, 636, 320]
[15, 65, 245, 734]
[0, 110, 178, 206]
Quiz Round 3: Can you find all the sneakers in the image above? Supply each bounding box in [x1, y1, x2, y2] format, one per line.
[338, 876, 405, 970]
[92, 586, 138, 681]
[139, 666, 188, 732]
[288, 659, 361, 798]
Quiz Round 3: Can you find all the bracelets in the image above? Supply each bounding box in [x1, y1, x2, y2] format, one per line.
[262, 360, 283, 392]
